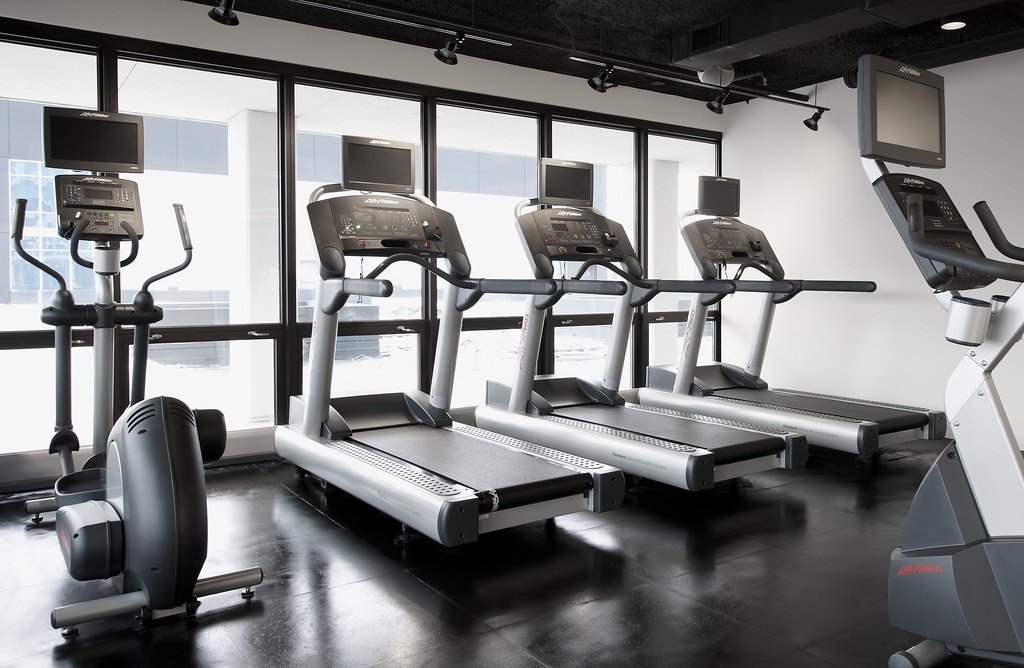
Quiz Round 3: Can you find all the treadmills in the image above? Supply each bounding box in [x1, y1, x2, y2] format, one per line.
[271, 132, 629, 612]
[848, 50, 1024, 668]
[646, 173, 949, 473]
[472, 155, 811, 527]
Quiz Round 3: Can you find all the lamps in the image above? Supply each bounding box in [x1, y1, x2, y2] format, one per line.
[207, 0, 240, 27]
[705, 87, 731, 115]
[433, 30, 466, 66]
[802, 109, 825, 131]
[588, 62, 614, 94]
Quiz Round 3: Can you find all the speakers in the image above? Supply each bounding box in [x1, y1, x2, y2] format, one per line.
[698, 64, 734, 87]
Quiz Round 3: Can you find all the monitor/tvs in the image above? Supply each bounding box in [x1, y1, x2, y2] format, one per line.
[539, 158, 593, 207]
[43, 107, 144, 174]
[342, 135, 415, 194]
[698, 176, 741, 218]
[858, 54, 946, 169]
